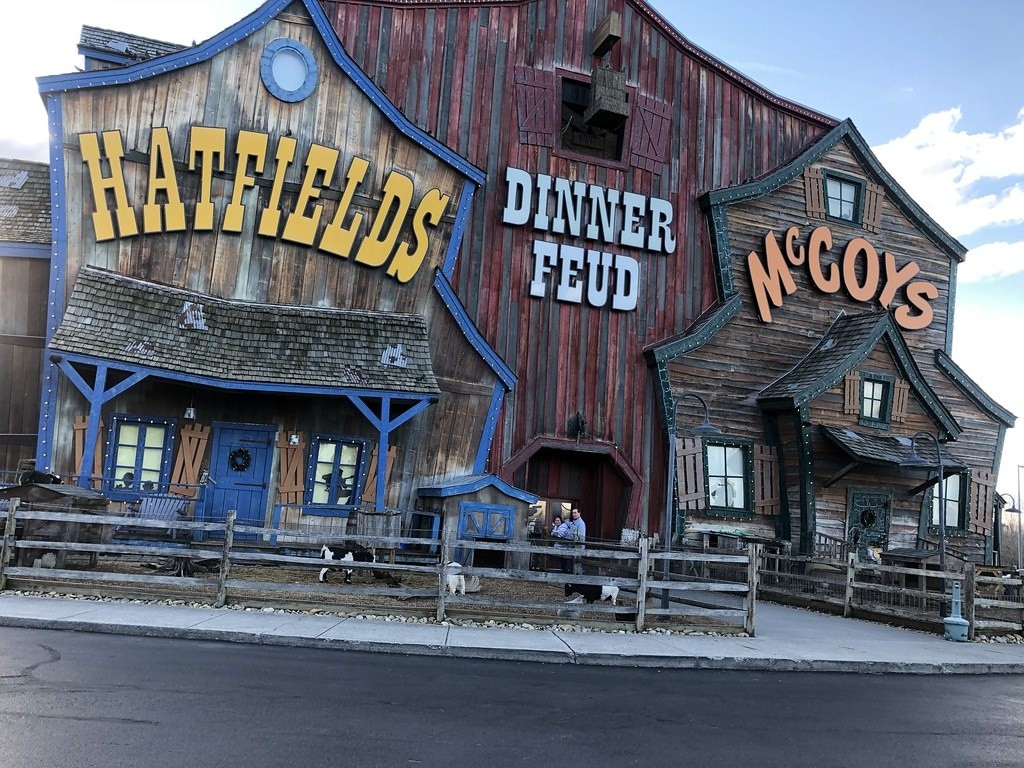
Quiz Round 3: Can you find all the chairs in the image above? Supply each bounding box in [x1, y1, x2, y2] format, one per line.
[112, 493, 192, 542]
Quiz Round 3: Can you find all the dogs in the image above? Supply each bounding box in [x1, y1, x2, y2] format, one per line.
[375, 571, 402, 586]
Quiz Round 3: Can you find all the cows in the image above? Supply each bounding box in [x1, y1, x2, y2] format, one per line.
[565, 582, 619, 606]
[436, 561, 465, 596]
[318, 542, 374, 584]
[19, 470, 65, 484]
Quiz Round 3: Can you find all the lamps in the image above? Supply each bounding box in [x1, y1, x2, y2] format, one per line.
[288, 411, 300, 446]
[183, 393, 196, 419]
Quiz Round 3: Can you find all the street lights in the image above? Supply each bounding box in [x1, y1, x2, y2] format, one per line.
[1017, 464, 1024, 567]
[657, 392, 725, 619]
[997, 493, 1022, 594]
[899, 430, 948, 618]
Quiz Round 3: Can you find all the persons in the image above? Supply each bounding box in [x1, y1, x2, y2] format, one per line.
[551, 507, 587, 572]
